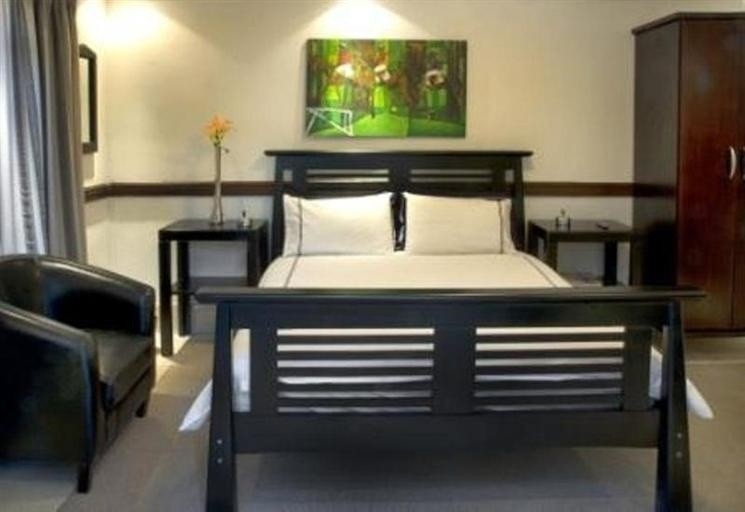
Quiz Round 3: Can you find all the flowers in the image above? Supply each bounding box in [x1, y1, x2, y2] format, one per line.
[205, 117, 235, 145]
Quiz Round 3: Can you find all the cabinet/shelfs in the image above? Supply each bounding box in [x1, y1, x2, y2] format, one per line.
[630, 9, 745, 334]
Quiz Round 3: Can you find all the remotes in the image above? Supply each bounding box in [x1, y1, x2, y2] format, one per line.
[596, 223, 609, 229]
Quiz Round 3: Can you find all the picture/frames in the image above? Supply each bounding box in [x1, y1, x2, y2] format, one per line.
[76, 42, 99, 153]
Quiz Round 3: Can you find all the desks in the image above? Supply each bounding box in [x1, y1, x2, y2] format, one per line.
[156, 217, 268, 357]
[528, 218, 635, 288]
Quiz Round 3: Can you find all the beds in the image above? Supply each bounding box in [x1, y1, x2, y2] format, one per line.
[198, 148, 692, 512]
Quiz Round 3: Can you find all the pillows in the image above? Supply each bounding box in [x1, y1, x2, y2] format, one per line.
[281, 191, 514, 256]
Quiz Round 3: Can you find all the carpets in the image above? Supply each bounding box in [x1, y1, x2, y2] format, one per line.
[59, 330, 745, 512]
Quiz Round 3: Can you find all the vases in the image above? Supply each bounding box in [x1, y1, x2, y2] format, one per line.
[211, 144, 224, 223]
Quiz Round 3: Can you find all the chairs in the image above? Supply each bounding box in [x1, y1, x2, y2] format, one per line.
[1, 245, 157, 495]
